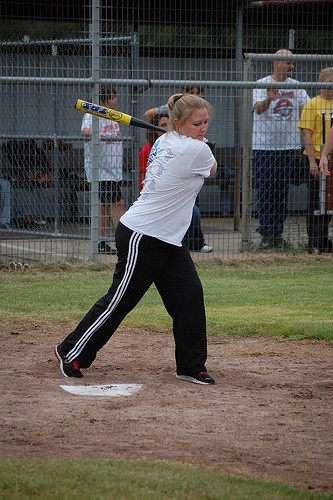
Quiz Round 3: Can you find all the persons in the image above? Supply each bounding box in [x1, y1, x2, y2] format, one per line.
[145, 76, 204, 208]
[53, 93, 217, 385]
[253, 49, 312, 250]
[138, 113, 213, 253]
[81, 85, 125, 254]
[297, 67, 333, 253]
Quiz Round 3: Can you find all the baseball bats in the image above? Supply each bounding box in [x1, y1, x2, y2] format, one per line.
[74, 97, 216, 151]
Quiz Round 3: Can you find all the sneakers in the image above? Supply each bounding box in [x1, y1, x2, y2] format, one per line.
[259, 237, 287, 252]
[308, 239, 332, 254]
[95, 241, 117, 255]
[200, 245, 213, 253]
[54, 345, 84, 378]
[176, 372, 215, 385]
